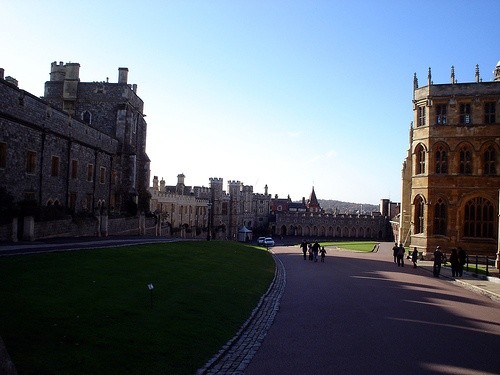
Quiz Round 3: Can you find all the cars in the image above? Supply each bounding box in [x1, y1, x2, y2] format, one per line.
[257, 237, 274, 247]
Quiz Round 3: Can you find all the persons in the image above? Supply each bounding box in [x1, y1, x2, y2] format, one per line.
[300, 240, 327, 263]
[449, 246, 467, 279]
[411, 247, 418, 269]
[392, 242, 405, 268]
[433, 246, 445, 278]
